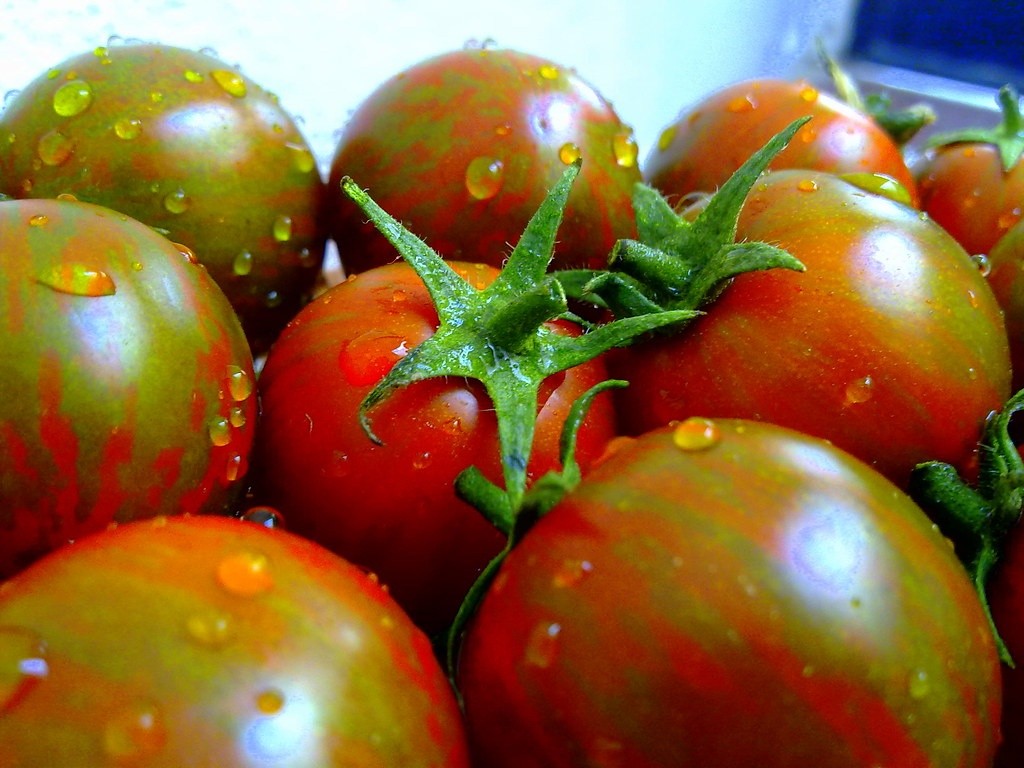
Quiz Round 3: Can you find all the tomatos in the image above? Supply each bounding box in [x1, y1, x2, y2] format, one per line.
[0, 47, 1024, 768]
